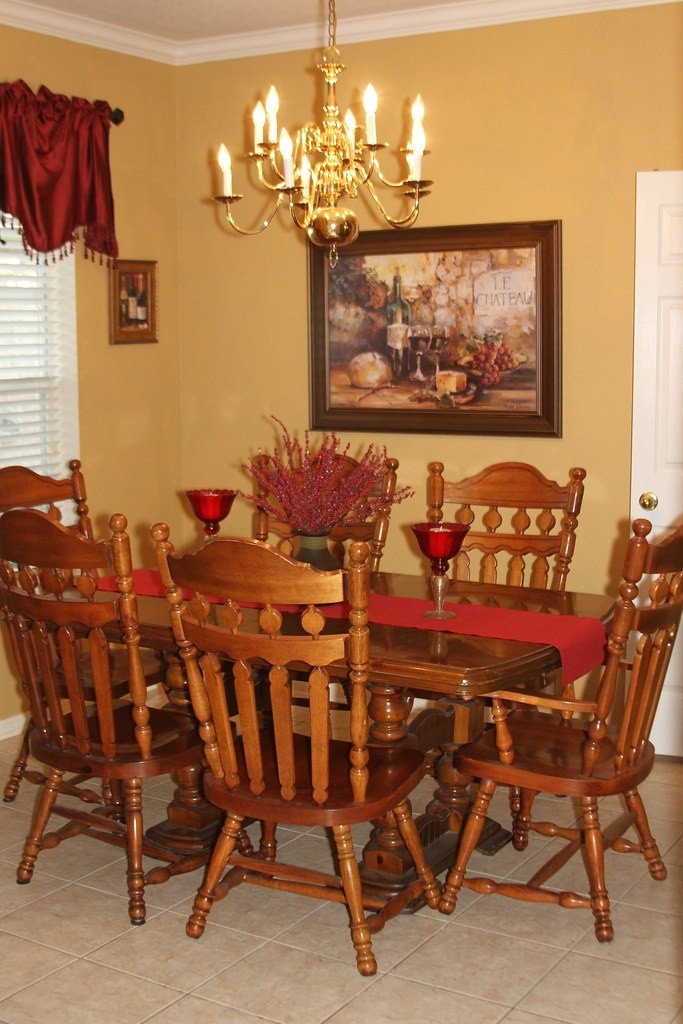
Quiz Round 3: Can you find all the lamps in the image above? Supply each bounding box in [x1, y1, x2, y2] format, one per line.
[212, 0, 434, 267]
[408, 522, 470, 619]
[184, 489, 238, 543]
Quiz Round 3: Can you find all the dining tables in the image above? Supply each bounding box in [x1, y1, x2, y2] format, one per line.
[24, 565, 616, 915]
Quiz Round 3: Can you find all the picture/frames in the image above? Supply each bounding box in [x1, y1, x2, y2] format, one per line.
[108, 258, 159, 345]
[307, 219, 562, 439]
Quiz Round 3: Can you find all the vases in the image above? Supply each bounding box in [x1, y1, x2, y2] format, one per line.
[292, 529, 340, 607]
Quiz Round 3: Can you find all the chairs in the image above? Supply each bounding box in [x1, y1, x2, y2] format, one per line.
[380, 460, 586, 824]
[152, 522, 443, 977]
[0, 459, 169, 834]
[0, 506, 258, 925]
[437, 519, 683, 943]
[253, 454, 399, 728]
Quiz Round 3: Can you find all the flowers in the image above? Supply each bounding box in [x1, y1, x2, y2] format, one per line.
[236, 414, 415, 530]
